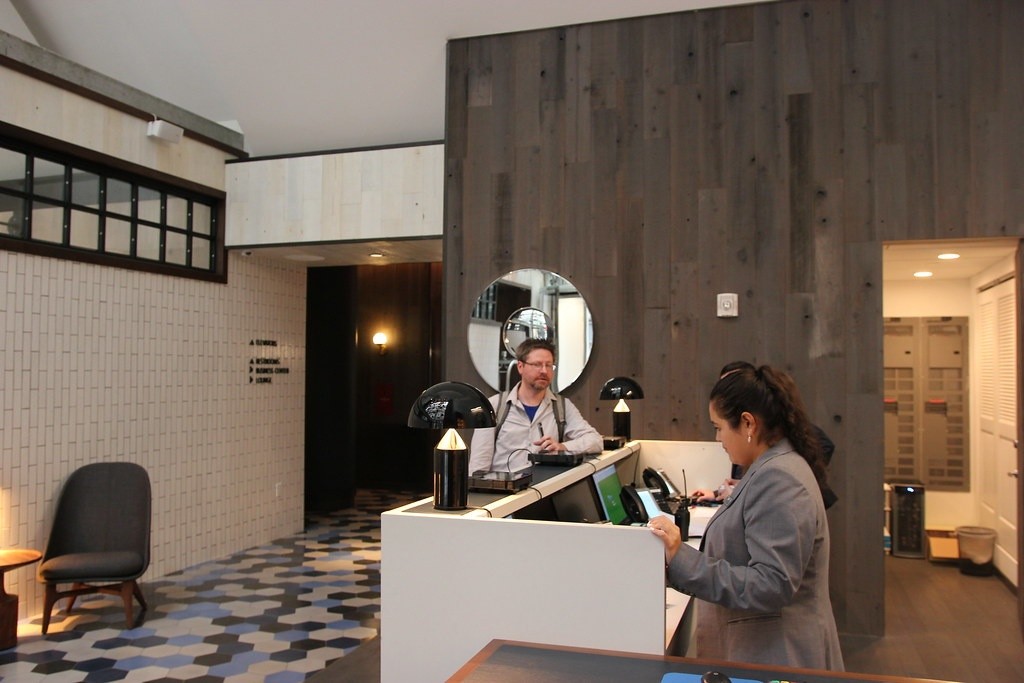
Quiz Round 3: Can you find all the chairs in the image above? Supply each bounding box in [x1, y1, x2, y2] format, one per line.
[34, 461, 152, 636]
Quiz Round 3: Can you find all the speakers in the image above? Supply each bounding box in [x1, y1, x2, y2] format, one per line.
[152, 120, 185, 144]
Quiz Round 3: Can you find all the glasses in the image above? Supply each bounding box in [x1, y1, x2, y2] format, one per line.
[524, 361, 556, 371]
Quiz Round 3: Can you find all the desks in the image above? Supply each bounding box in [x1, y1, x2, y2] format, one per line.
[0, 548, 40, 650]
[440, 640, 957, 682]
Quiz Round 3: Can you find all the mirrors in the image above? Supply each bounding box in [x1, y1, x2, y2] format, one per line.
[465, 266, 595, 398]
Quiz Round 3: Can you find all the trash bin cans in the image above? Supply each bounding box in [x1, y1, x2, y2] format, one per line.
[956, 525, 998, 577]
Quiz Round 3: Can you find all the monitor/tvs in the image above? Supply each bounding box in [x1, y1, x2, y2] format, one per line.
[587, 464, 632, 526]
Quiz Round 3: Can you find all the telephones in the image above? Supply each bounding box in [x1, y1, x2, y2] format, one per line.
[619, 485, 650, 524]
[643, 466, 681, 502]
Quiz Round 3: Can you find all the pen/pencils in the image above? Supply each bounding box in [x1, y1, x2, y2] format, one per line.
[538, 422, 544, 437]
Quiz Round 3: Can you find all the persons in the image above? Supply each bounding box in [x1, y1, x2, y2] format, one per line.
[450, 412, 465, 428]
[646, 359, 845, 671]
[469, 338, 604, 476]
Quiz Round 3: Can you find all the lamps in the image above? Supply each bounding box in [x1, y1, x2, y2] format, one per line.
[407, 380, 498, 509]
[598, 376, 644, 442]
